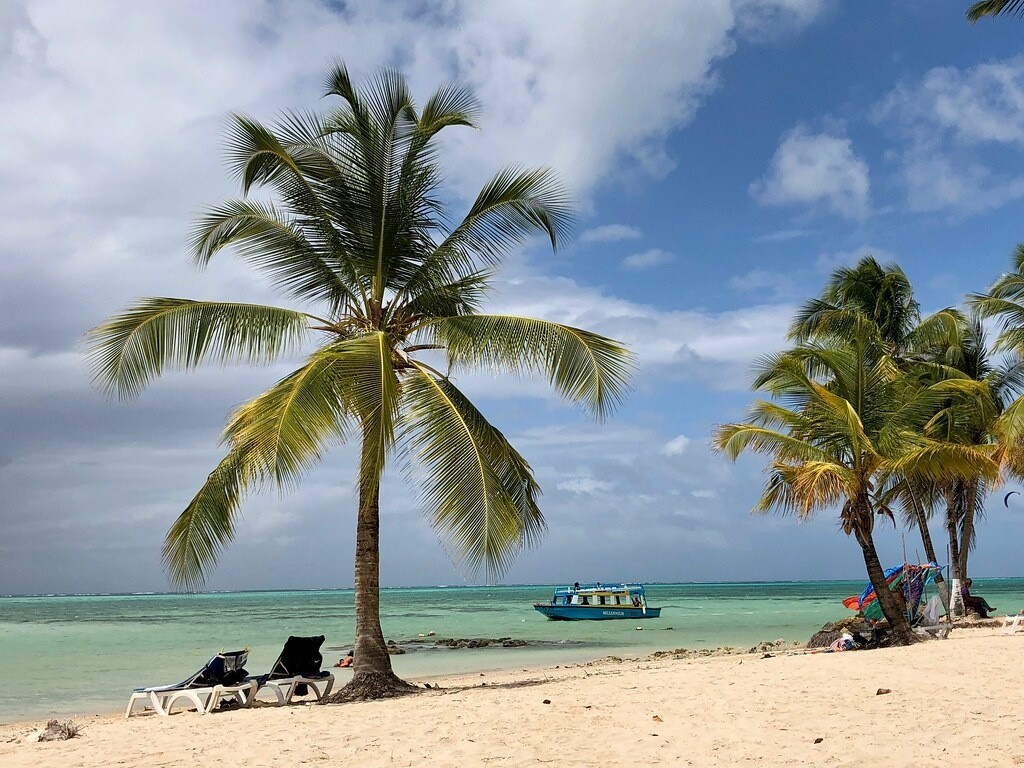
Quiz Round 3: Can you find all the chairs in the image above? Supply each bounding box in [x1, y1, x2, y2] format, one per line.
[910, 597, 946, 628]
[205, 635, 335, 711]
[124, 645, 258, 719]
[1001, 614, 1024, 635]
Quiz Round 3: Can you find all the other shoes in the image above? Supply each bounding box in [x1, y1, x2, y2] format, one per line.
[653, 716, 663, 722]
[220, 698, 239, 711]
[877, 689, 889, 695]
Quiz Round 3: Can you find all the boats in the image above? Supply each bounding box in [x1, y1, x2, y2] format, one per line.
[533, 580, 662, 621]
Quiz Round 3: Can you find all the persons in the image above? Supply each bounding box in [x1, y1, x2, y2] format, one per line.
[631, 596, 641, 607]
[961, 578, 997, 619]
[334, 649, 354, 668]
